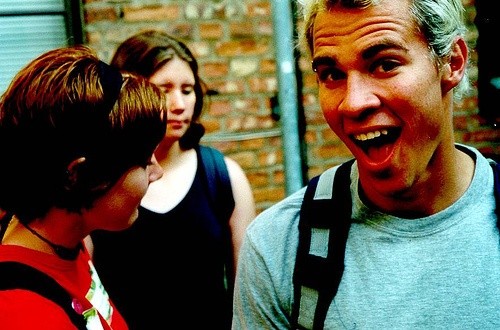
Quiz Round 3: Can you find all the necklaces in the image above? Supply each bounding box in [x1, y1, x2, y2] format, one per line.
[14, 213, 84, 261]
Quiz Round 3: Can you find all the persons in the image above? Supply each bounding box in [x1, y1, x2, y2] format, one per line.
[0, 46, 169, 330]
[228, 0, 500, 329]
[86, 30, 255, 329]
